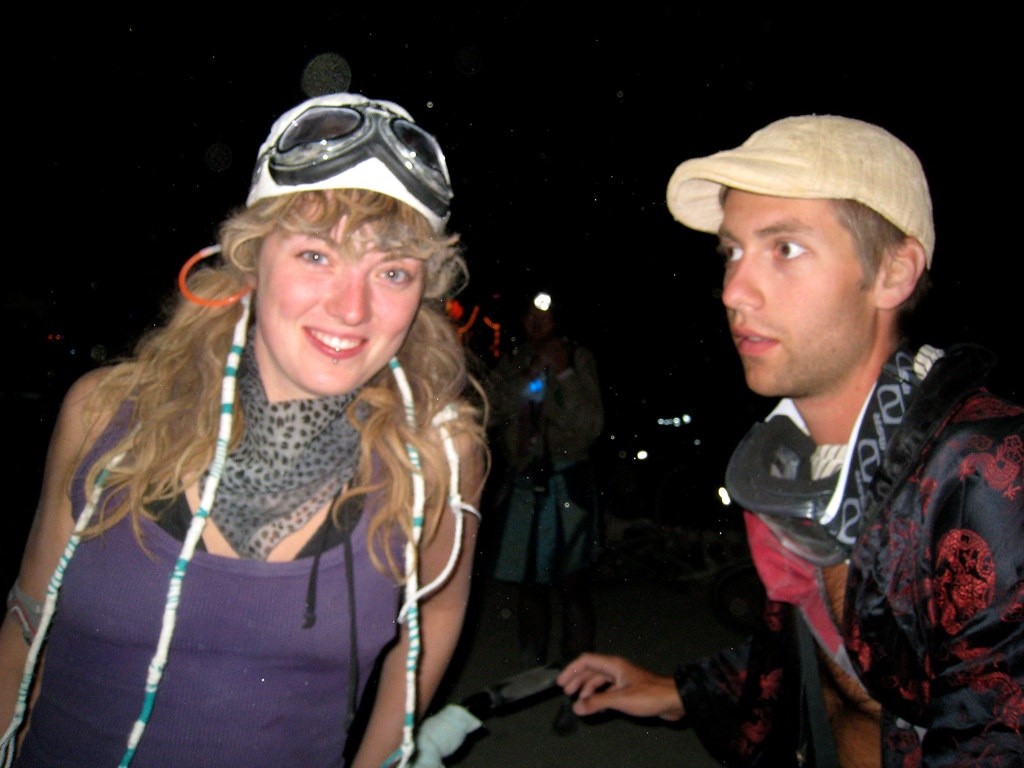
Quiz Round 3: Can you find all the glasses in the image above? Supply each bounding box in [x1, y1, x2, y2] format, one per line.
[724, 414, 851, 569]
[265, 103, 452, 217]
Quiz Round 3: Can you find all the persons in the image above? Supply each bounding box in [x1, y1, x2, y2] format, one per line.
[0, 92, 492, 768]
[466, 292, 602, 583]
[555, 115, 1024, 768]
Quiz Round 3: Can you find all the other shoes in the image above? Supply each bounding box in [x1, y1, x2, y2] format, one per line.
[553, 696, 581, 735]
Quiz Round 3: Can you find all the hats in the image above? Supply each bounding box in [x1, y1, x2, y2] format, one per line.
[666, 114, 936, 273]
[247, 93, 452, 237]
